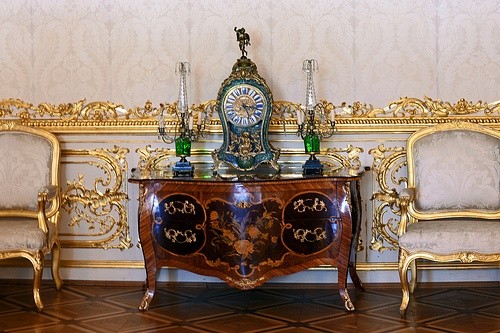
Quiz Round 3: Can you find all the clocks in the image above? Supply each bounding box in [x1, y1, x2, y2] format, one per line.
[212, 27, 283, 171]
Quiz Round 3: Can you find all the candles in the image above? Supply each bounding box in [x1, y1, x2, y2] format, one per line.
[197, 111, 202, 124]
[187, 116, 194, 129]
[157, 113, 166, 132]
[296, 109, 305, 126]
[329, 109, 335, 125]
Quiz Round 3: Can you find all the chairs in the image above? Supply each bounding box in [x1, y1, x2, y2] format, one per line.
[0, 120, 65, 311]
[397, 121, 500, 310]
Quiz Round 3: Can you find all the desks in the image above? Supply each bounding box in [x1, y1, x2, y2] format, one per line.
[126, 162, 366, 312]
